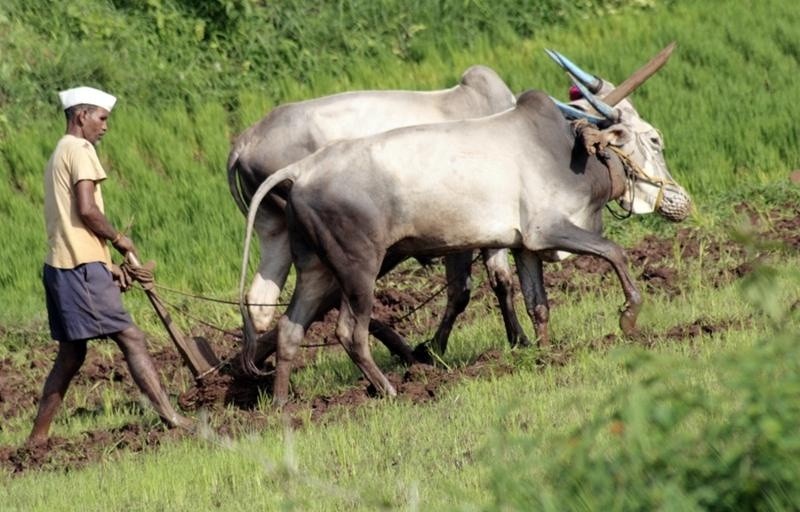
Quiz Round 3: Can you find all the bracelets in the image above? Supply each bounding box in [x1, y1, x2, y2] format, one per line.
[111, 232, 121, 244]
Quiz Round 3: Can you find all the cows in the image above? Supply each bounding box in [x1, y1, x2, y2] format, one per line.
[237, 68, 693, 404]
[224, 48, 641, 368]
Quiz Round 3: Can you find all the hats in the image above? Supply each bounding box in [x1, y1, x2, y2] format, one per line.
[58, 86, 117, 112]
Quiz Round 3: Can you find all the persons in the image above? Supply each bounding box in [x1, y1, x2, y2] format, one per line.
[28, 86, 192, 448]
